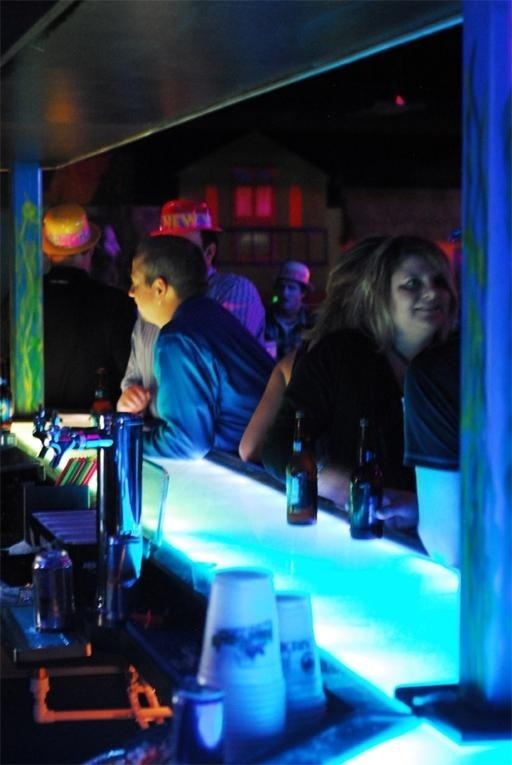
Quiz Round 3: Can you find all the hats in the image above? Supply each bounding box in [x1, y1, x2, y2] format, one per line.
[43, 202, 102, 256]
[148, 198, 223, 237]
[276, 262, 315, 293]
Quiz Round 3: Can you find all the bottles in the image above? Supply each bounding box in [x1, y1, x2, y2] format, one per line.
[90, 366, 113, 437]
[0, 359, 13, 429]
[283, 410, 318, 526]
[348, 412, 384, 542]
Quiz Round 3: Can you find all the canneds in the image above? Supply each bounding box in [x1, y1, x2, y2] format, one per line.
[31, 549, 73, 634]
[170, 674, 225, 764]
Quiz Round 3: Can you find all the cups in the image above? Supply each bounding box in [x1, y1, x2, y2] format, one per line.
[195, 557, 327, 765]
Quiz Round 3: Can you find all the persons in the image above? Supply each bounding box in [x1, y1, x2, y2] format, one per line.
[259, 231, 460, 532]
[116, 197, 264, 421]
[343, 314, 462, 566]
[262, 260, 324, 365]
[118, 232, 288, 497]
[238, 236, 386, 470]
[40, 201, 138, 414]
